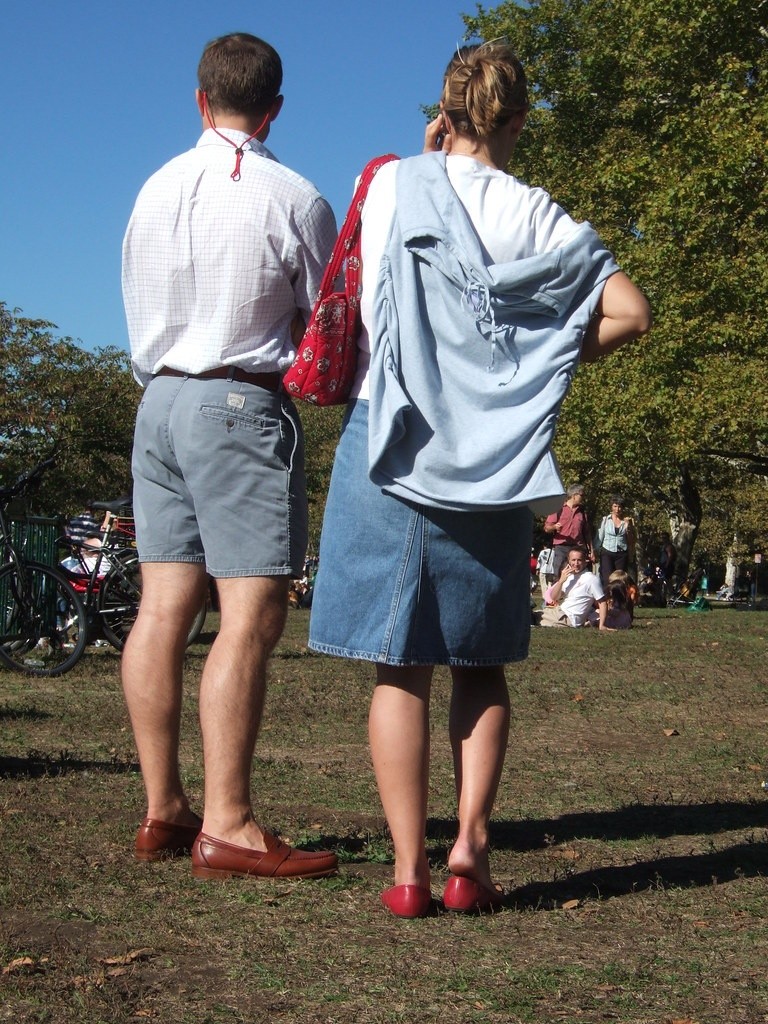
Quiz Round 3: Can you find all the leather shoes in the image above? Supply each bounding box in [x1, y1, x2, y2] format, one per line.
[134, 812, 204, 862]
[192, 830, 340, 880]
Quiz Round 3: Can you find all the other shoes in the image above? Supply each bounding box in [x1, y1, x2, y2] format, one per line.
[444, 875, 505, 912]
[381, 885, 431, 918]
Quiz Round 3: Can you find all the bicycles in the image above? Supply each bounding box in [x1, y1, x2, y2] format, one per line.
[0, 459, 210, 678]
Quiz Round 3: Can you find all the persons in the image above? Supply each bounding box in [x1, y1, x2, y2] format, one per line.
[305, 551, 319, 578]
[304, 44, 653, 918]
[120, 31, 340, 883]
[530, 483, 679, 632]
[717, 584, 732, 600]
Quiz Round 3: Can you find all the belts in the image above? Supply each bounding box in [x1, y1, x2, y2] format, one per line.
[153, 366, 282, 391]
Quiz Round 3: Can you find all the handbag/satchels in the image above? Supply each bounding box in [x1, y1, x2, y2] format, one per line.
[282, 153, 400, 407]
